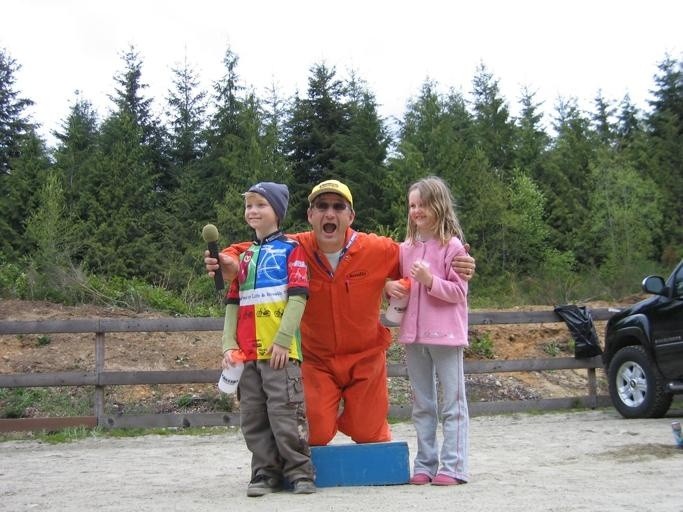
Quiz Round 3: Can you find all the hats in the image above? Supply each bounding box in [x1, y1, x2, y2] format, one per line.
[248, 181, 290, 220]
[308, 180, 353, 210]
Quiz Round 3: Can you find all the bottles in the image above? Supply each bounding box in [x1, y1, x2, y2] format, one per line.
[218, 349, 247, 394]
[385, 277, 411, 324]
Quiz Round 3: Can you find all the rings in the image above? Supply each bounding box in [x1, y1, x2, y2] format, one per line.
[466, 268, 471, 274]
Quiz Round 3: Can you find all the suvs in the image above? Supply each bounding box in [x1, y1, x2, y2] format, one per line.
[600, 259, 682, 419]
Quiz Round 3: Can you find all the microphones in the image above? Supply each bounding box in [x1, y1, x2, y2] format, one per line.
[202, 224, 224, 289]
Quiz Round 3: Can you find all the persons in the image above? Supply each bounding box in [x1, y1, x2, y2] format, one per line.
[383, 172, 471, 487]
[203, 180, 476, 446]
[220, 180, 318, 498]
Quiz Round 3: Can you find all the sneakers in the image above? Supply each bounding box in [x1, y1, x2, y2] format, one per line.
[431, 474, 461, 487]
[411, 473, 430, 485]
[247, 475, 283, 496]
[292, 478, 316, 494]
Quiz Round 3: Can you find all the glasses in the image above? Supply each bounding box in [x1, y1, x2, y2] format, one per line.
[310, 201, 351, 211]
